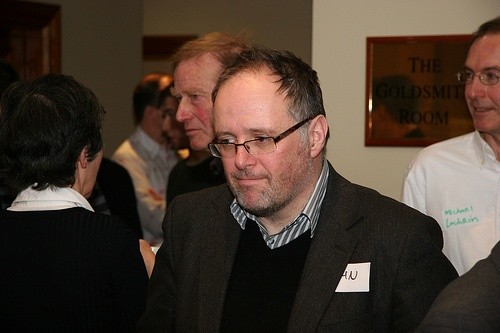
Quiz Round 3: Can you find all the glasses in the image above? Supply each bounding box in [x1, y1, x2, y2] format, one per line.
[456, 69, 500, 86]
[208, 115, 312, 159]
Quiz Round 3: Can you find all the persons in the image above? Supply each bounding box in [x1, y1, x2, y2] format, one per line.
[372, 73, 426, 138]
[401, 17, 500, 277]
[164, 32, 245, 209]
[0, 72, 156, 333]
[137, 48, 459, 333]
[0, 64, 182, 247]
[414, 239, 500, 333]
